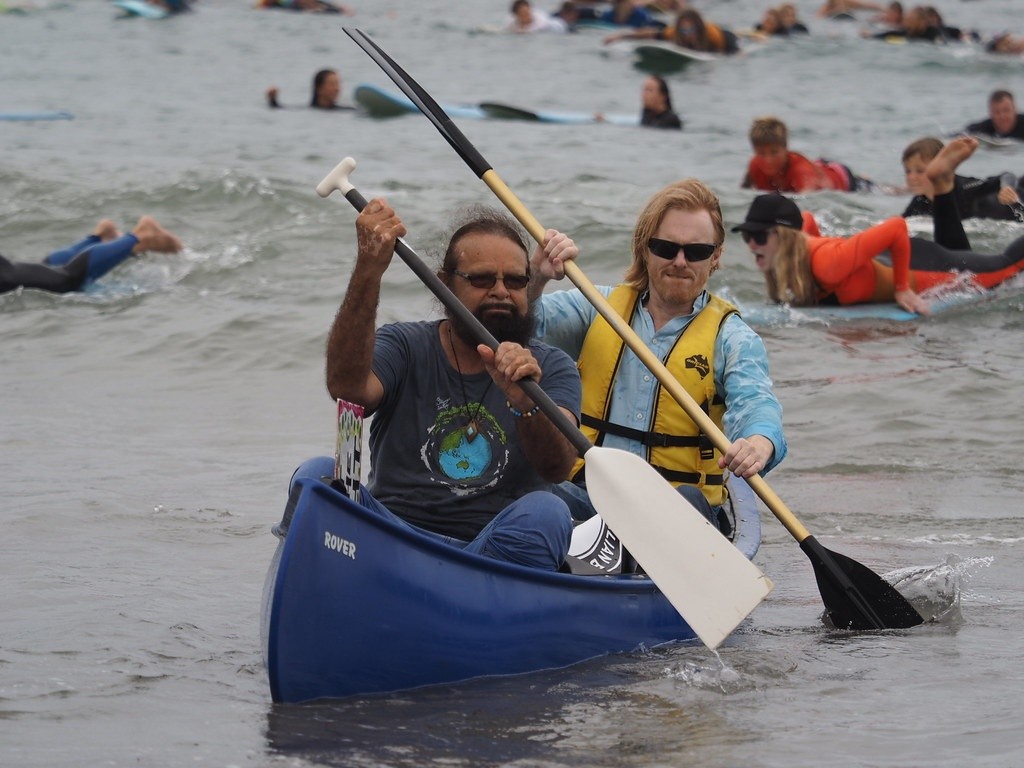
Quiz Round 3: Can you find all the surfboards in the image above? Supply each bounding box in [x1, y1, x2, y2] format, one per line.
[111, 0, 167, 19]
[745, 291, 990, 326]
[570, 23, 618, 35]
[633, 35, 783, 64]
[349, 81, 479, 121]
[477, 100, 638, 126]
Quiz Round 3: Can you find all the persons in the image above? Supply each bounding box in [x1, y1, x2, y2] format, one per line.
[0, 215, 185, 294]
[491, 0, 1024, 57]
[733, 136, 1024, 315]
[528, 178, 787, 532]
[267, 69, 354, 111]
[957, 90, 1024, 140]
[591, 76, 685, 131]
[896, 138, 1024, 220]
[739, 117, 912, 196]
[116, 0, 352, 21]
[324, 201, 582, 569]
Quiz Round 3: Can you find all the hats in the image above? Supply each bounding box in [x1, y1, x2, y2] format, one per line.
[731, 193, 804, 234]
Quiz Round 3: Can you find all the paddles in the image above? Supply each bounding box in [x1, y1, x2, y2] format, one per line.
[314, 150, 779, 657]
[341, 19, 926, 632]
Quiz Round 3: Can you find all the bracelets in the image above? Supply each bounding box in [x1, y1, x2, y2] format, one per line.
[505, 398, 541, 418]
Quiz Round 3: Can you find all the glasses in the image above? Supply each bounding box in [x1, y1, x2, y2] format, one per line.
[454, 270, 531, 290]
[742, 230, 776, 245]
[648, 238, 717, 261]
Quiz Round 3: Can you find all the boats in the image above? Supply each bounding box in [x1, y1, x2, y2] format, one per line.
[260, 457, 763, 704]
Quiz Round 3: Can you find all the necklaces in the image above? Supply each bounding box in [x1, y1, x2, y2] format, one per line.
[448, 324, 493, 443]
